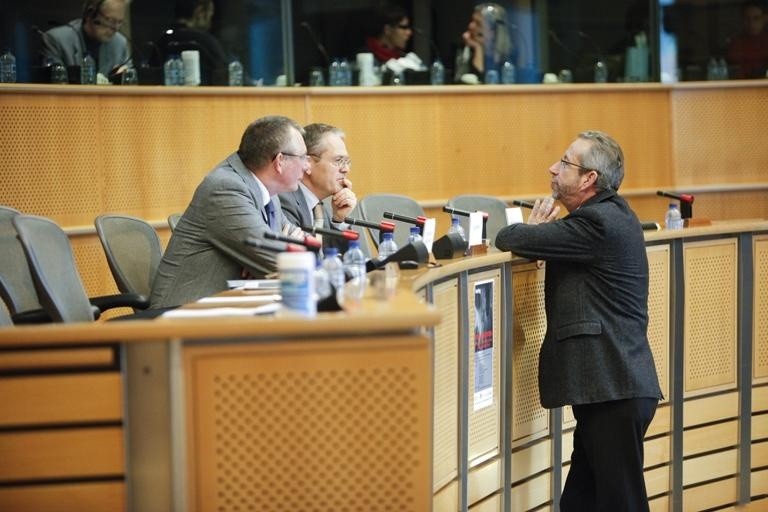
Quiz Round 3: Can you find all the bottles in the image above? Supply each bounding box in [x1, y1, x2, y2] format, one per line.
[594, 61, 607, 83]
[501, 60, 518, 84]
[323, 248, 345, 289]
[343, 241, 366, 276]
[665, 203, 684, 228]
[405, 226, 424, 246]
[430, 56, 445, 85]
[378, 233, 399, 262]
[316, 259, 332, 299]
[228, 56, 244, 87]
[708, 53, 729, 80]
[559, 68, 575, 84]
[164, 54, 185, 86]
[329, 55, 352, 86]
[447, 218, 467, 242]
[0, 47, 16, 83]
[81, 50, 97, 85]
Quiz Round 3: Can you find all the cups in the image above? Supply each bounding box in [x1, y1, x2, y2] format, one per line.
[50, 62, 70, 83]
[484, 70, 500, 84]
[307, 66, 324, 86]
[121, 68, 138, 84]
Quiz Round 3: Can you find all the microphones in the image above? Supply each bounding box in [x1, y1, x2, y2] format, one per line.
[46, 18, 85, 59]
[260, 230, 324, 251]
[441, 205, 489, 221]
[382, 210, 429, 229]
[300, 224, 359, 245]
[478, 32, 510, 63]
[343, 216, 396, 235]
[299, 19, 333, 64]
[244, 236, 309, 253]
[655, 188, 682, 203]
[93, 18, 140, 84]
[511, 199, 536, 211]
[495, 17, 518, 49]
[545, 28, 567, 51]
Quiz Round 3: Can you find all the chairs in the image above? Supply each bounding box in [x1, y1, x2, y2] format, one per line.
[0, 206, 150, 323]
[15, 17, 45, 83]
[95, 215, 163, 314]
[360, 194, 426, 254]
[321, 196, 372, 260]
[168, 212, 184, 232]
[295, 21, 331, 67]
[140, 40, 165, 68]
[10, 214, 180, 323]
[167, 43, 212, 85]
[447, 195, 511, 248]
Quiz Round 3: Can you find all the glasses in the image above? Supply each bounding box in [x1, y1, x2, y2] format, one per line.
[559, 158, 591, 173]
[310, 152, 352, 167]
[273, 151, 307, 168]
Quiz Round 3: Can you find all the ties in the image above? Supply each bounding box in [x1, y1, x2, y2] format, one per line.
[265, 197, 276, 230]
[313, 202, 325, 229]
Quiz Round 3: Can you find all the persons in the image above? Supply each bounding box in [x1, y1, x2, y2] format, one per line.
[495, 131, 664, 512]
[278, 123, 358, 262]
[454, 2, 517, 84]
[149, 0, 226, 87]
[348, 9, 414, 85]
[41, 0, 138, 85]
[724, 0, 768, 80]
[147, 116, 306, 310]
